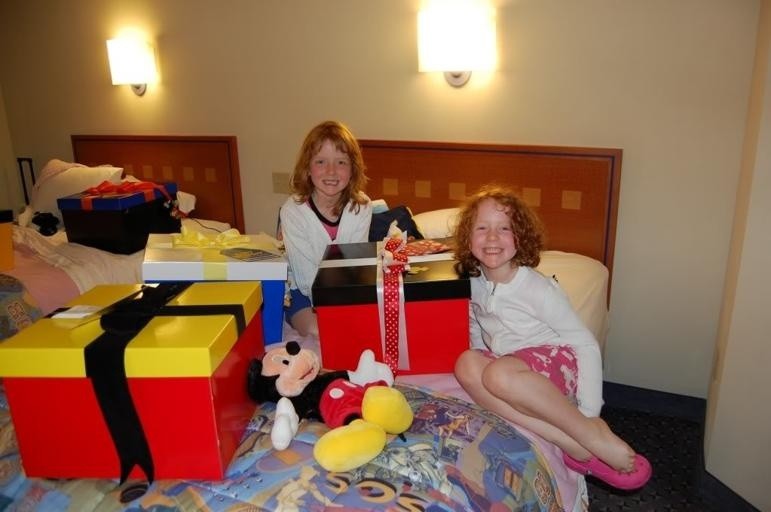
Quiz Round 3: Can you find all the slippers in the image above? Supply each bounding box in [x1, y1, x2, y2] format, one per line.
[563, 452, 652, 490]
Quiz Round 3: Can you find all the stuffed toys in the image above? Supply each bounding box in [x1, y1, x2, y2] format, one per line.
[247, 341, 414, 473]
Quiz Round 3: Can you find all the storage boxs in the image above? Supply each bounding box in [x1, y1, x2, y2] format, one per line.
[0, 280, 265, 482]
[143, 227, 292, 343]
[62, 182, 183, 258]
[313, 242, 472, 379]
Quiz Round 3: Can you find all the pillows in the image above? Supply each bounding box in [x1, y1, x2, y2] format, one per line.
[32, 159, 125, 229]
[125, 174, 197, 215]
[405, 208, 478, 241]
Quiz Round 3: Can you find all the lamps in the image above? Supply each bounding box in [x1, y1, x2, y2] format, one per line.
[103, 28, 158, 96]
[417, 2, 500, 87]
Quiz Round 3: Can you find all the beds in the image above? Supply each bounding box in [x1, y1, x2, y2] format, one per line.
[0, 135, 626, 512]
[0, 129, 247, 343]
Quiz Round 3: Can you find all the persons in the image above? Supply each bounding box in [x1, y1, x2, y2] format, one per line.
[279, 120, 372, 339]
[455, 188, 653, 491]
